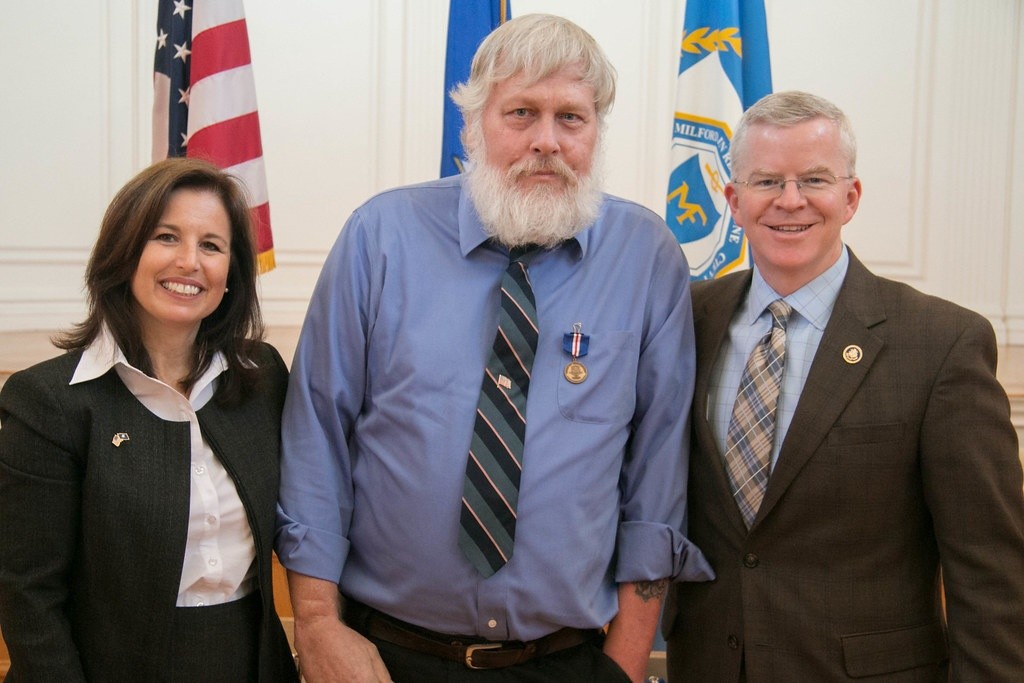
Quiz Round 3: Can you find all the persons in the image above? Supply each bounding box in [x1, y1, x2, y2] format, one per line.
[1, 160, 301, 683]
[274, 12, 714, 683]
[661, 93, 1024, 683]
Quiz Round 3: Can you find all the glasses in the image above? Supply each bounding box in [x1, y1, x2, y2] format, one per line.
[733, 173, 855, 193]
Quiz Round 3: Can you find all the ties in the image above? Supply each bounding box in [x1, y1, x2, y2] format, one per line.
[455, 246, 539, 581]
[722, 299, 795, 533]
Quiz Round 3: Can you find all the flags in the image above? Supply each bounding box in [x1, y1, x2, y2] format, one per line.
[152, 0, 276, 279]
[440, 0, 514, 179]
[666, 0, 775, 284]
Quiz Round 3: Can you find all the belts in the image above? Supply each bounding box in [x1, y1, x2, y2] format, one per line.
[362, 615, 591, 668]
